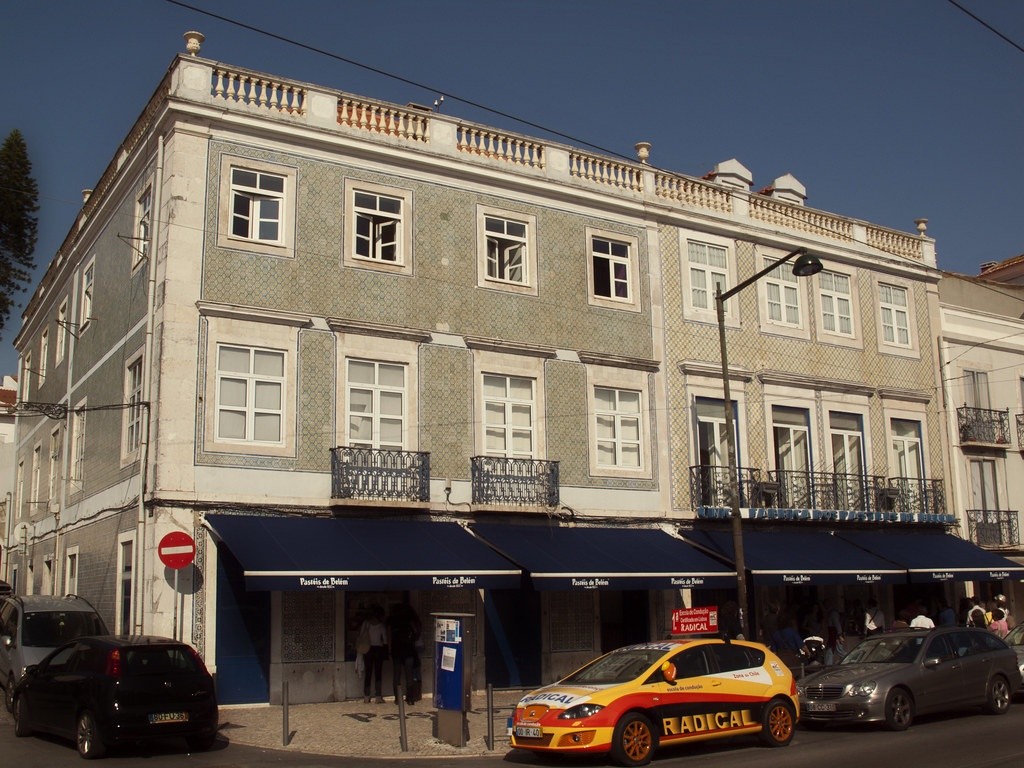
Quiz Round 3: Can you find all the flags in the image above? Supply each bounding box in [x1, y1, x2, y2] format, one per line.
[834, 532, 1024, 583]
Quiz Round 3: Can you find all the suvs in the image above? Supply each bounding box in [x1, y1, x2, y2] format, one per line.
[795, 624, 1022, 731]
[0, 593, 113, 707]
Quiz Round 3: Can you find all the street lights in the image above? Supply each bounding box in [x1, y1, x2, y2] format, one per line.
[714, 243, 825, 640]
[5, 490, 13, 583]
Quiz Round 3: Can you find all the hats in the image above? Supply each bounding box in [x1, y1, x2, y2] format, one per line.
[994, 594, 1006, 602]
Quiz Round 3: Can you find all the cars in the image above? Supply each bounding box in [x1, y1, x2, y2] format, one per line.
[12, 634, 218, 761]
[0, 579, 13, 608]
[508, 639, 800, 767]
[1004, 622, 1024, 701]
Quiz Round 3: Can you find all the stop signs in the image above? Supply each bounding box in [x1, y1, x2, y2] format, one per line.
[159, 531, 196, 570]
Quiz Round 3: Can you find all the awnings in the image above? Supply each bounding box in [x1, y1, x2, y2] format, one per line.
[676, 525, 908, 585]
[464, 522, 738, 592]
[201, 513, 522, 592]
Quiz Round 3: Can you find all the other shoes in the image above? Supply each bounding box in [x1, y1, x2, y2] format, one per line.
[405, 699, 414, 705]
[364, 696, 371, 703]
[394, 700, 399, 705]
[375, 696, 386, 703]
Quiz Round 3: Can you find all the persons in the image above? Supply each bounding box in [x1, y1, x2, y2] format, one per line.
[383, 595, 422, 705]
[360, 606, 388, 703]
[764, 594, 1010, 679]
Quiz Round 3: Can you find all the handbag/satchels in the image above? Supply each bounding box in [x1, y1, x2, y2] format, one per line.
[863, 627, 868, 635]
[356, 620, 370, 654]
